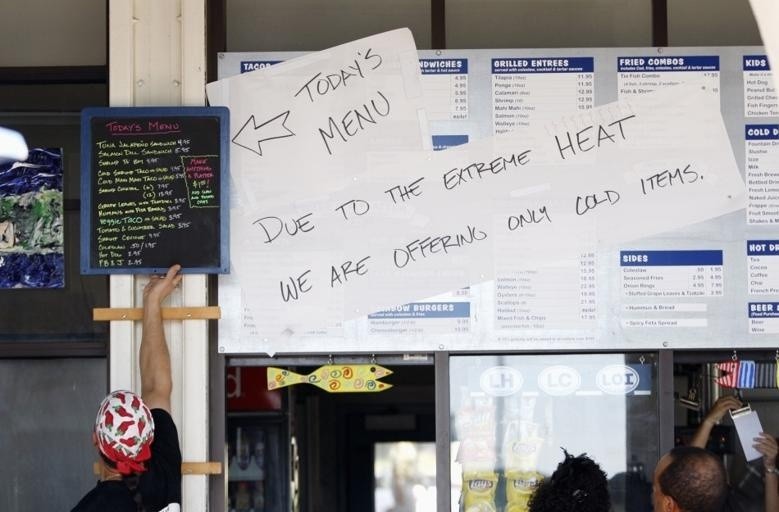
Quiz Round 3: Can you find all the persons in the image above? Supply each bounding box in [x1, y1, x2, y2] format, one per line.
[69, 263, 182, 512]
[525, 445, 611, 511]
[650, 446, 728, 511]
[689, 394, 779, 512]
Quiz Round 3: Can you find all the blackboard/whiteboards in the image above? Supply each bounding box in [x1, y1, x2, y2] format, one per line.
[0, 109, 109, 360]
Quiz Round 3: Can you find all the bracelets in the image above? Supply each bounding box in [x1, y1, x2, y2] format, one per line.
[764, 463, 779, 474]
